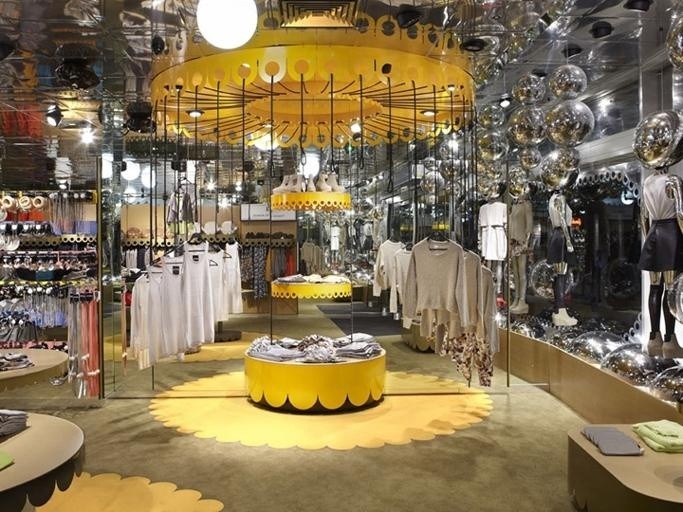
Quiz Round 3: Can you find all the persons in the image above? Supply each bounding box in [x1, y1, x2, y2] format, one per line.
[636, 168, 683, 360]
[547, 191, 578, 326]
[477, 193, 508, 293]
[509, 198, 534, 314]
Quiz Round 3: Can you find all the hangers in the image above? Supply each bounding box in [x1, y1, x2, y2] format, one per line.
[385, 224, 489, 269]
[136, 231, 240, 279]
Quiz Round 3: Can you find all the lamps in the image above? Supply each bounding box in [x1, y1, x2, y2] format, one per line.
[46, 105, 64, 127]
[185, 108, 204, 118]
[498, 95, 512, 109]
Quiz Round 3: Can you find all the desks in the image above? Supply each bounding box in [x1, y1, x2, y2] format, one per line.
[0, 409, 86, 512]
[0, 348, 69, 390]
[567, 423, 683, 511]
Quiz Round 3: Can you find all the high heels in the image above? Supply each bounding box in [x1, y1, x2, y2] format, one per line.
[510, 299, 529, 314]
[648, 332, 663, 356]
[662, 334, 683, 359]
[552, 308, 578, 326]
[497, 298, 507, 307]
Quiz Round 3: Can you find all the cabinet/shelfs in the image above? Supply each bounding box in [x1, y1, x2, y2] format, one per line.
[267, 157, 353, 345]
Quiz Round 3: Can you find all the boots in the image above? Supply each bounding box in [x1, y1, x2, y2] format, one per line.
[272, 174, 346, 192]
